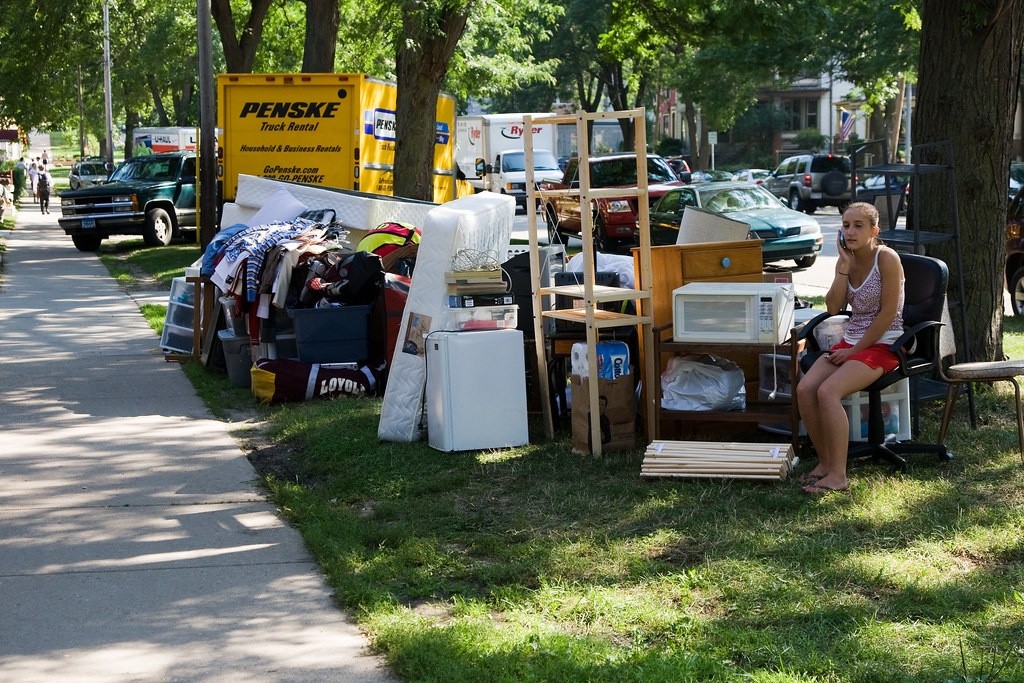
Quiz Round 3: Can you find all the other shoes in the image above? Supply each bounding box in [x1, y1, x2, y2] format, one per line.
[42, 212, 45, 214]
[46, 209, 50, 214]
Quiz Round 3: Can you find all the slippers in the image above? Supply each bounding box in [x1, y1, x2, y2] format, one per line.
[802, 482, 850, 494]
[799, 472, 823, 484]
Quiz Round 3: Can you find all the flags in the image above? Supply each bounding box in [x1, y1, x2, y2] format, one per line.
[839, 107, 857, 143]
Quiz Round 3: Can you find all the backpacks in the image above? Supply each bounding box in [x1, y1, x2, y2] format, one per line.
[36, 171, 50, 196]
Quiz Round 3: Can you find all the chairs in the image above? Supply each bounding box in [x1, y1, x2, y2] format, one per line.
[795, 255, 953, 471]
[935, 294, 1024, 464]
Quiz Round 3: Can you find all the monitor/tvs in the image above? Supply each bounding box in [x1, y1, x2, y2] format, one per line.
[500, 245, 567, 336]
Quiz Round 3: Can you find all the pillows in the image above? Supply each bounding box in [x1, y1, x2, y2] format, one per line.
[248, 184, 308, 228]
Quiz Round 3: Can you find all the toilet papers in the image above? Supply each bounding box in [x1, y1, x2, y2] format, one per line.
[570, 341, 631, 382]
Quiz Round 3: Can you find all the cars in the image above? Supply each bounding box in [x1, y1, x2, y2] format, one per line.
[691, 169, 734, 184]
[632, 181, 824, 268]
[853, 160, 1024, 323]
[735, 168, 771, 185]
[68, 160, 117, 191]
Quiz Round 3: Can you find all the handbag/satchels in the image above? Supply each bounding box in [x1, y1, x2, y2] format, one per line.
[814, 313, 850, 352]
[657, 354, 747, 412]
[250, 357, 385, 404]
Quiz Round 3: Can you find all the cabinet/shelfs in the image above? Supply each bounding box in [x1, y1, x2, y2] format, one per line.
[630, 238, 766, 417]
[652, 319, 816, 455]
[756, 353, 912, 441]
[160, 275, 205, 355]
[851, 139, 978, 435]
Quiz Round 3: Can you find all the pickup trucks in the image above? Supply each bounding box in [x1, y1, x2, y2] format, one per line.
[539, 150, 686, 256]
[56, 148, 218, 252]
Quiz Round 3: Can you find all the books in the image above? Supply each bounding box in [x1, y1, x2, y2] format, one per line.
[444, 270, 508, 296]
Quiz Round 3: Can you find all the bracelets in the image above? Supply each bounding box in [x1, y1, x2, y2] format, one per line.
[839, 272, 850, 275]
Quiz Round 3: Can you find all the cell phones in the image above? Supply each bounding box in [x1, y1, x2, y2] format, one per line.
[840, 227, 844, 248]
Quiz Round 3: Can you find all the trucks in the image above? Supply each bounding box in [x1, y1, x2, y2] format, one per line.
[216, 72, 487, 209]
[131, 127, 219, 157]
[455, 113, 564, 215]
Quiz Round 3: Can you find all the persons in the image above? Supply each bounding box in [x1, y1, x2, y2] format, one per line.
[16, 150, 53, 215]
[797, 202, 905, 492]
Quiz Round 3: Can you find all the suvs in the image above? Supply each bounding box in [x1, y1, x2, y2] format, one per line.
[663, 154, 694, 184]
[759, 154, 854, 212]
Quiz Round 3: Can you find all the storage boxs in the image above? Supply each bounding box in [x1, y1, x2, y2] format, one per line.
[286, 305, 370, 364]
[216, 331, 252, 388]
[218, 298, 239, 335]
[447, 306, 518, 331]
[570, 374, 634, 455]
